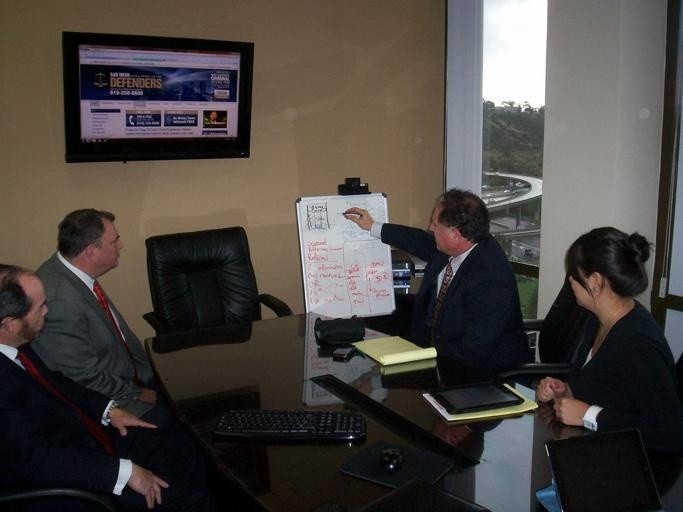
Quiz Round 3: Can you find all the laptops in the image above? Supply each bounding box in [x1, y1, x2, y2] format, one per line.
[545, 426, 663, 512]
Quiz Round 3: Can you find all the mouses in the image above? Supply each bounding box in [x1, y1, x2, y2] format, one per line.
[376, 444, 410, 473]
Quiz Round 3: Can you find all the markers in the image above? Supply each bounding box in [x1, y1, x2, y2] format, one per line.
[337, 212, 362, 218]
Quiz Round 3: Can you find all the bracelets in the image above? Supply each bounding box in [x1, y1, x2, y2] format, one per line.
[103, 403, 118, 419]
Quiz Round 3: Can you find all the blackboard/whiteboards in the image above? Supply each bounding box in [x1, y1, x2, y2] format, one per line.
[295, 192, 396, 319]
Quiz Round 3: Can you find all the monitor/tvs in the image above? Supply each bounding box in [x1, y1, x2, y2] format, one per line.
[62, 30, 256, 163]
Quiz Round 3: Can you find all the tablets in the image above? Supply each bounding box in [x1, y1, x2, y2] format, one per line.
[430, 381, 525, 415]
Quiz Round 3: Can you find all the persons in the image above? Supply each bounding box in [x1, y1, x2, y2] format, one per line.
[0, 262, 173, 508]
[342, 186, 532, 367]
[25, 208, 157, 408]
[208, 111, 219, 123]
[532, 225, 683, 512]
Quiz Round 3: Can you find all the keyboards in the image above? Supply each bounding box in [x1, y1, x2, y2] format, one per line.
[212, 410, 367, 446]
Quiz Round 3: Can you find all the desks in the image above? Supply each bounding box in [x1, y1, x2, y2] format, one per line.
[136, 311, 683, 511]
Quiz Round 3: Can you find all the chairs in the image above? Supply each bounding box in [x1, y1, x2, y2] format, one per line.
[500, 268, 599, 390]
[0, 481, 124, 512]
[140, 224, 295, 336]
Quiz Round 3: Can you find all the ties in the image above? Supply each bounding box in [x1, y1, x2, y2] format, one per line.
[429, 261, 454, 347]
[16, 350, 114, 453]
[92, 280, 138, 381]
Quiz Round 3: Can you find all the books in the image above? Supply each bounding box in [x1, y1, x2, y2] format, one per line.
[362, 354, 438, 376]
[420, 382, 539, 422]
[349, 335, 439, 367]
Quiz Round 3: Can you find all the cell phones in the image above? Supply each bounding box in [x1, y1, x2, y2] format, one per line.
[333, 348, 353, 358]
